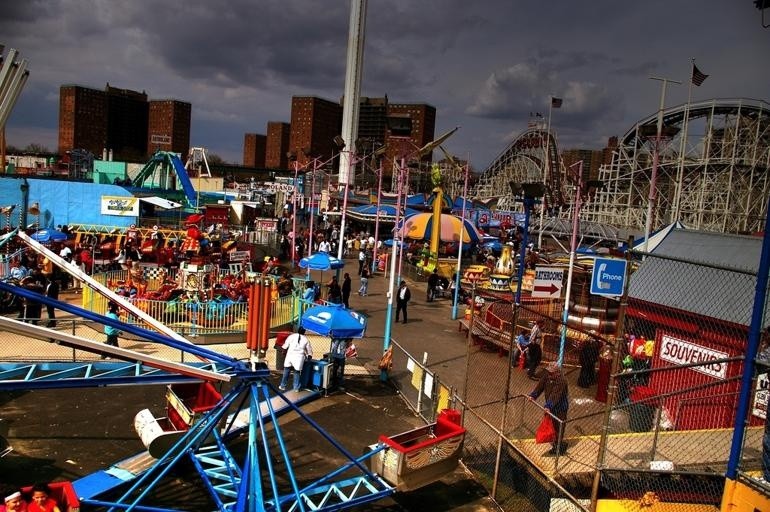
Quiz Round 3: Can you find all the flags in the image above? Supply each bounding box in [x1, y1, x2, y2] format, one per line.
[552, 96, 563, 107]
[691, 64, 709, 87]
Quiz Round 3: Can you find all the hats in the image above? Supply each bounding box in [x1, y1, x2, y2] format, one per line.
[544, 361, 560, 373]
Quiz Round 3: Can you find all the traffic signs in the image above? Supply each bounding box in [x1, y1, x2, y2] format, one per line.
[531, 266, 564, 299]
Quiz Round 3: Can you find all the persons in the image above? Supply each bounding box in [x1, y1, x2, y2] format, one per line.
[1, 219, 547, 391]
[578, 329, 601, 387]
[530, 362, 568, 455]
[27, 483, 60, 512]
[0, 485, 27, 512]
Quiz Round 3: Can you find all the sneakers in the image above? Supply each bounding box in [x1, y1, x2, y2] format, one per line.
[278, 384, 289, 392]
[293, 388, 301, 393]
[547, 441, 570, 457]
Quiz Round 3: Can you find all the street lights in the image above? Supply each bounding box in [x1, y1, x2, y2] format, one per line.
[285, 136, 422, 382]
[489, 179, 546, 497]
[452, 149, 472, 320]
[636, 123, 682, 262]
[556, 159, 604, 370]
